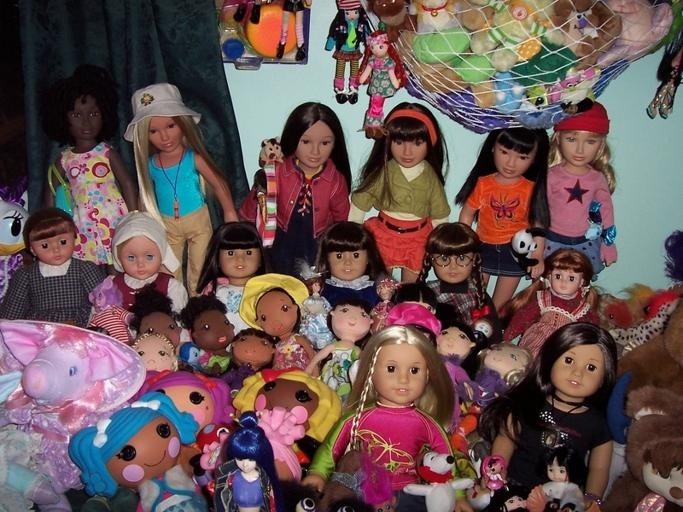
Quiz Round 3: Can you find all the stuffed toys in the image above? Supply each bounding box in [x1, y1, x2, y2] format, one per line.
[369, 1, 673, 136]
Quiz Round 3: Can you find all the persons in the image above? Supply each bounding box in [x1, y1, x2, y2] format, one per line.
[239, 101, 353, 281]
[325, 0, 371, 106]
[357, 30, 407, 141]
[349, 101, 452, 283]
[277, 0, 307, 61]
[647, 32, 683, 120]
[456, 126, 552, 312]
[40, 65, 139, 265]
[1, 0, 682, 512]
[1, 211, 682, 512]
[123, 83, 238, 298]
[542, 102, 617, 279]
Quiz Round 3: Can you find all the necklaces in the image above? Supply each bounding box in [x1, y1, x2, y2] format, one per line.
[159, 150, 184, 222]
